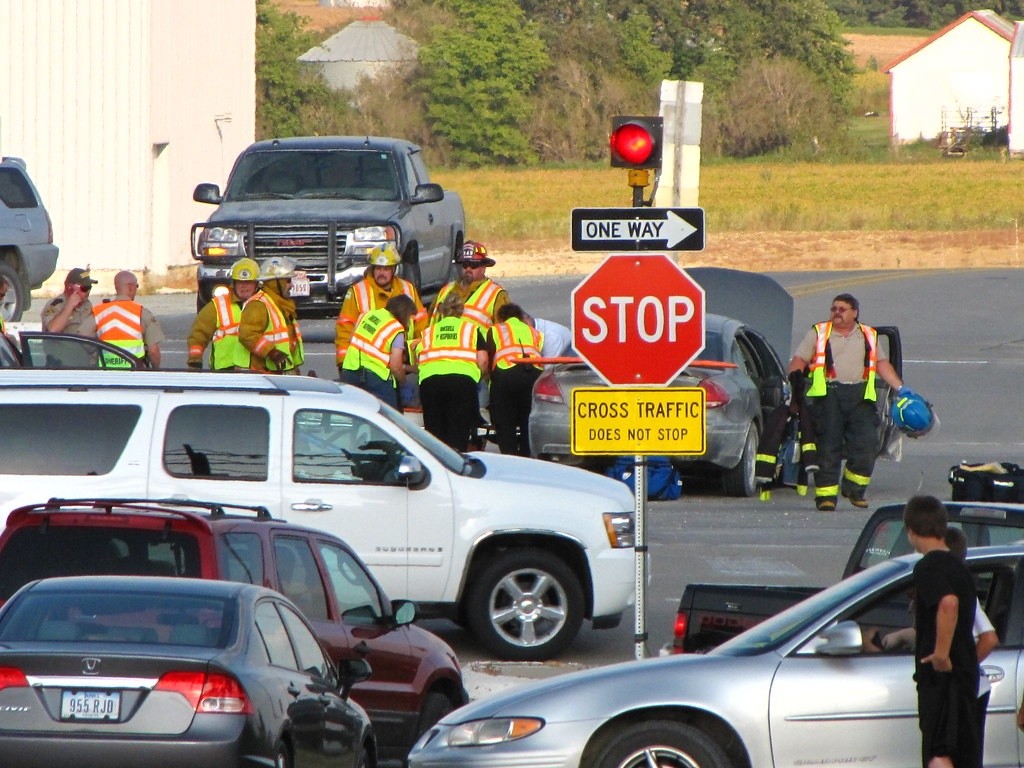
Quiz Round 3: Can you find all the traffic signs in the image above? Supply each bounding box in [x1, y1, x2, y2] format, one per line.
[571, 207, 705, 252]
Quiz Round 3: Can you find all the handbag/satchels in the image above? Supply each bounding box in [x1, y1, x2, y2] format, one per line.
[606, 453, 682, 501]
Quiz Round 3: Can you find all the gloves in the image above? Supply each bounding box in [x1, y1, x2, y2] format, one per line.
[269, 348, 292, 372]
[898, 387, 913, 396]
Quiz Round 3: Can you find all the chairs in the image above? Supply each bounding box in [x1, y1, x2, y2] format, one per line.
[1001, 596, 1024, 649]
[54, 537, 178, 578]
[39, 620, 211, 648]
[260, 155, 394, 192]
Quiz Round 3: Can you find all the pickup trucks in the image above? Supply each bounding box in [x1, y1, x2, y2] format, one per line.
[190, 135, 466, 315]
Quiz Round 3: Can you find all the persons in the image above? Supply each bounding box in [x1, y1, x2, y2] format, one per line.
[786, 292, 903, 510]
[91, 270, 163, 369]
[337, 294, 543, 456]
[40, 267, 98, 369]
[333, 243, 429, 366]
[185, 258, 261, 372]
[237, 257, 305, 376]
[881, 496, 998, 768]
[0, 274, 11, 338]
[428, 239, 509, 327]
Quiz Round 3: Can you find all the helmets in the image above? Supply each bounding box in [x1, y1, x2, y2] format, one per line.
[255, 257, 298, 280]
[891, 393, 933, 434]
[451, 240, 495, 267]
[229, 257, 259, 281]
[366, 242, 402, 265]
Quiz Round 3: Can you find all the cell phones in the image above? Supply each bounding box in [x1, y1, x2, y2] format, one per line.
[66, 283, 82, 308]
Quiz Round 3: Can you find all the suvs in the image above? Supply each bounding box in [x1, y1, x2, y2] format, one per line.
[0, 497, 469, 768]
[0, 156, 60, 322]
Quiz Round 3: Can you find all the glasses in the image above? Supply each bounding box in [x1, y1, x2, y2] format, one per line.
[0, 293, 5, 297]
[462, 261, 483, 269]
[830, 307, 853, 312]
[74, 284, 91, 292]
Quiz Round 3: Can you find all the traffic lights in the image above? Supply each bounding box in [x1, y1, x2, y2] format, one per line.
[610, 114, 664, 169]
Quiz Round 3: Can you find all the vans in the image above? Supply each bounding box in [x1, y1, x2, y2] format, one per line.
[1, 369, 639, 662]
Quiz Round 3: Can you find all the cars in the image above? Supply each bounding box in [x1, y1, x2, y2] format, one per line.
[510, 267, 902, 497]
[396, 332, 636, 657]
[408, 501, 1024, 768]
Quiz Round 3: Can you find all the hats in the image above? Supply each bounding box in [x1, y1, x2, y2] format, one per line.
[66, 268, 98, 287]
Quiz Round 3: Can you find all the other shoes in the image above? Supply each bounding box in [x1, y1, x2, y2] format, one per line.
[841, 492, 869, 508]
[818, 500, 835, 510]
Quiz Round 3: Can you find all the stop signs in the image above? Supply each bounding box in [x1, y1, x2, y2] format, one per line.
[570, 254, 706, 387]
